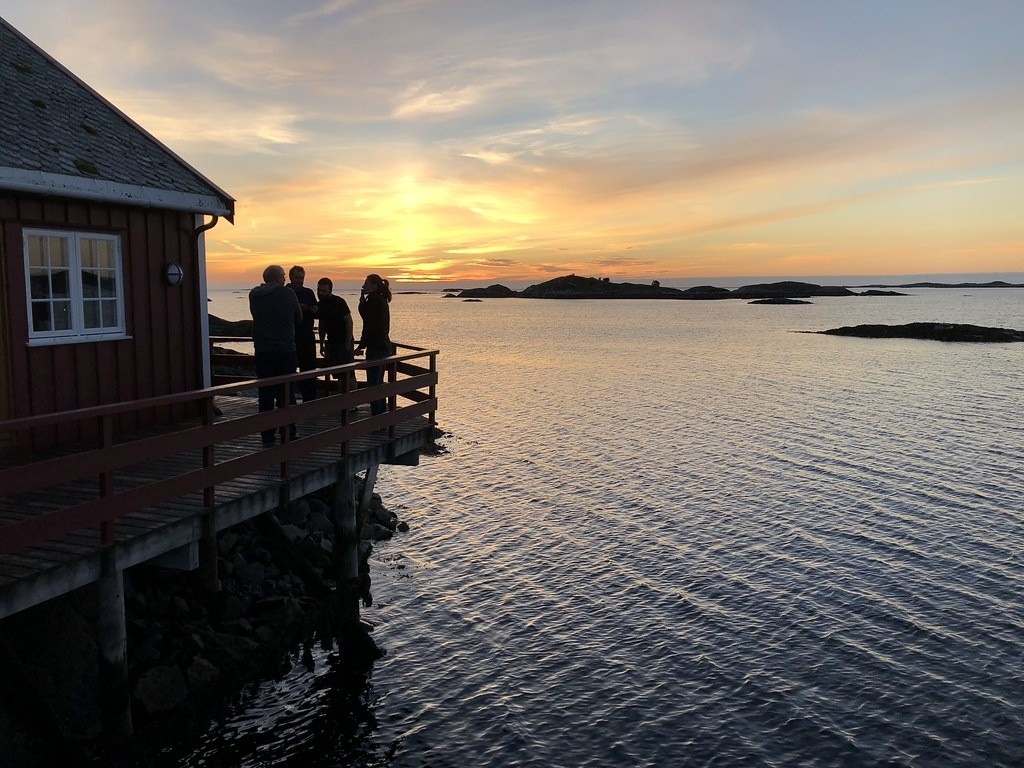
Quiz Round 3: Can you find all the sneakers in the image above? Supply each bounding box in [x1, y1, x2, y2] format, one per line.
[348, 404, 359, 411]
[262, 435, 276, 448]
[290, 434, 301, 441]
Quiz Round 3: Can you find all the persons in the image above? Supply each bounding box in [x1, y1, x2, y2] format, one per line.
[354, 274, 392, 432]
[249, 266, 358, 448]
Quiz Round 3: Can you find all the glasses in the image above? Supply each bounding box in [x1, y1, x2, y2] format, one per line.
[281, 274, 286, 278]
